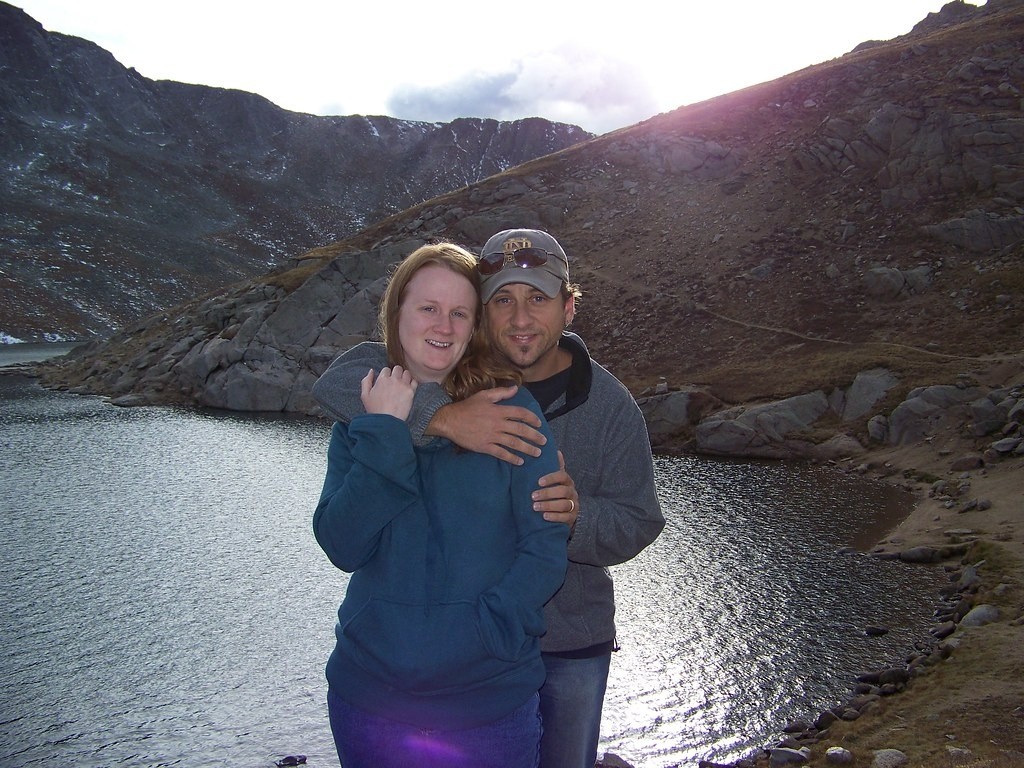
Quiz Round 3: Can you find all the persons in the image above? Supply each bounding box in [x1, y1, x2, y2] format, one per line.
[313, 243, 572, 768]
[313, 230, 667, 768]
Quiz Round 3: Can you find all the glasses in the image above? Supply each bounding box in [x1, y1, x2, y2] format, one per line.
[478, 247, 567, 275]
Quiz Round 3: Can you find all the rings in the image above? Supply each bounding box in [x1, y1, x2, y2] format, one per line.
[567, 498, 574, 513]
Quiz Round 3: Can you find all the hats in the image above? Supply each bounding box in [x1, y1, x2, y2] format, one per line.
[475, 228, 569, 304]
